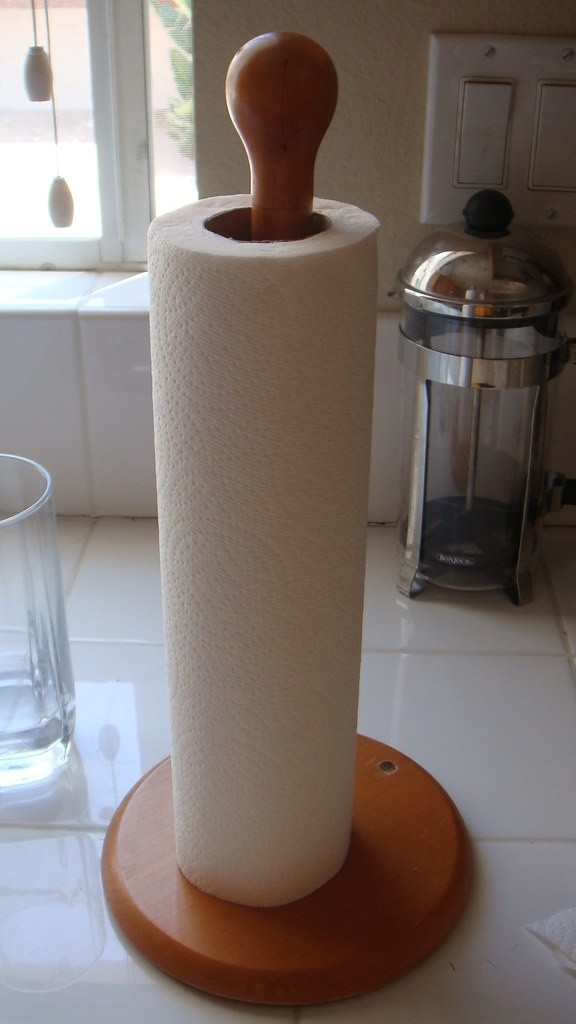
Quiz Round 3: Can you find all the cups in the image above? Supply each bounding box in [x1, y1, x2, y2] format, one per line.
[0, 454, 76, 787]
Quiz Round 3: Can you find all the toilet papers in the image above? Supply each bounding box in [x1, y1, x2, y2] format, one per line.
[145, 189, 381, 913]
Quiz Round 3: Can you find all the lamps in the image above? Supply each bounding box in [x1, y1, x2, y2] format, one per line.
[28, 0, 75, 228]
